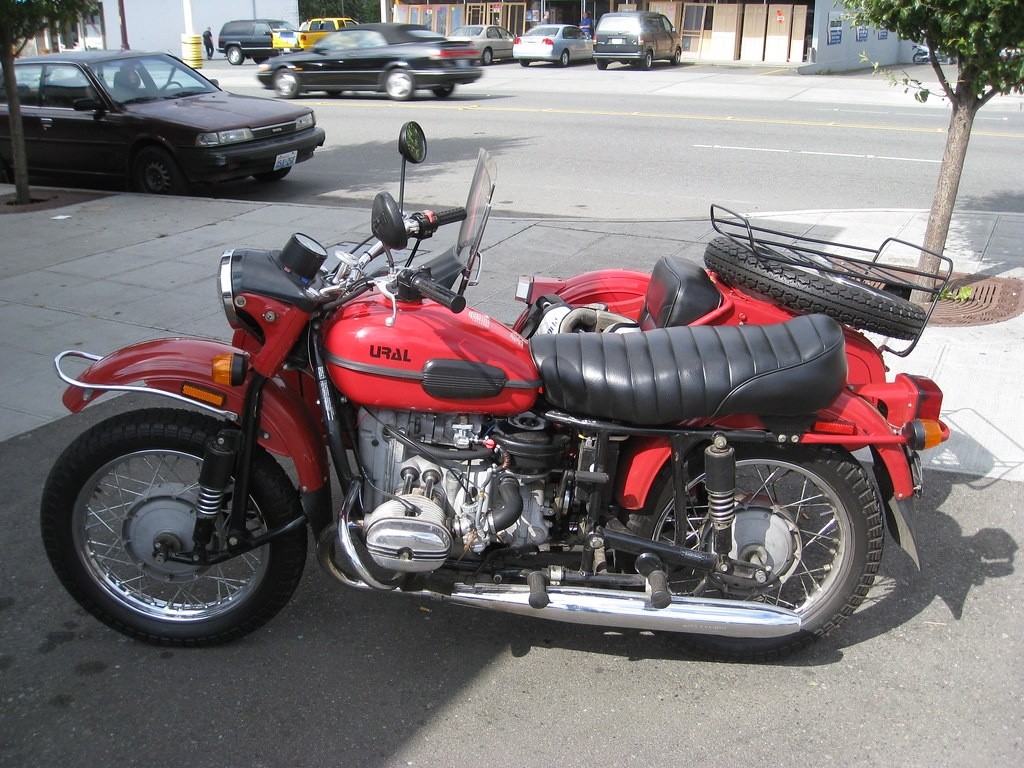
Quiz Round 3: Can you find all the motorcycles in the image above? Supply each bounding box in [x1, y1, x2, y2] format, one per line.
[911, 42, 956, 65]
[35, 119, 957, 664]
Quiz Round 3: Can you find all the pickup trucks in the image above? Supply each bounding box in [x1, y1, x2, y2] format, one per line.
[271, 17, 360, 56]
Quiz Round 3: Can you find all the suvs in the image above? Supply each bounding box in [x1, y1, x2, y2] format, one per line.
[215, 19, 298, 65]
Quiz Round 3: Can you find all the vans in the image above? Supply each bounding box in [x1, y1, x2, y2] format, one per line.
[592, 11, 683, 72]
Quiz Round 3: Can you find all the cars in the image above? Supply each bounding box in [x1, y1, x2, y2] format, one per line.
[513, 23, 594, 68]
[256, 22, 484, 103]
[0, 48, 327, 195]
[444, 24, 518, 67]
